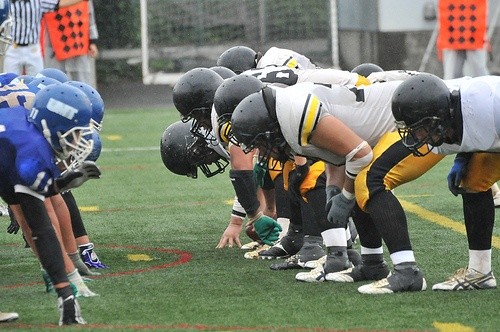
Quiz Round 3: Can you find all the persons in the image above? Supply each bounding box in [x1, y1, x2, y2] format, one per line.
[161, 46, 500, 294]
[0, 1, 110, 324]
[391, 73, 500, 291]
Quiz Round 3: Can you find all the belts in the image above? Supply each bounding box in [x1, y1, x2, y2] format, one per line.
[16, 43, 28, 46]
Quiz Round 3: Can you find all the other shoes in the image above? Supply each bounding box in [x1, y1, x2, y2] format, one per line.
[0, 312, 19, 322]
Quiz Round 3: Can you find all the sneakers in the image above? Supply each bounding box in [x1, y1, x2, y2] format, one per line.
[240, 240, 496, 294]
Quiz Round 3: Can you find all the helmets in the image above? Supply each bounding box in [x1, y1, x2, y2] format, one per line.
[78, 127, 102, 162]
[27, 77, 62, 95]
[209, 66, 237, 79]
[214, 74, 264, 122]
[63, 80, 105, 132]
[350, 63, 385, 78]
[29, 83, 93, 164]
[172, 66, 225, 144]
[39, 68, 69, 82]
[160, 120, 229, 178]
[231, 86, 292, 171]
[390, 73, 454, 156]
[217, 45, 261, 75]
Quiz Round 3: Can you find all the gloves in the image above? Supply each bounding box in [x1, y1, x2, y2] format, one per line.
[249, 210, 282, 246]
[288, 162, 309, 202]
[52, 161, 102, 195]
[325, 185, 357, 227]
[447, 153, 470, 197]
[6, 207, 112, 325]
[253, 156, 268, 189]
